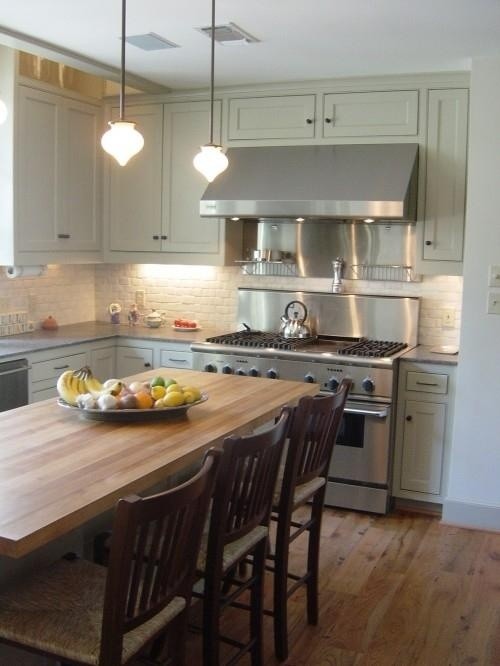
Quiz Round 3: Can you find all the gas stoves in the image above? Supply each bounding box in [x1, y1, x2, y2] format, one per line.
[190, 329, 415, 404]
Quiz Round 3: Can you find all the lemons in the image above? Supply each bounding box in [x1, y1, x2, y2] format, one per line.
[151, 384, 202, 408]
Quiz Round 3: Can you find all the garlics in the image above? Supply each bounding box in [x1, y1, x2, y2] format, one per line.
[76, 379, 151, 410]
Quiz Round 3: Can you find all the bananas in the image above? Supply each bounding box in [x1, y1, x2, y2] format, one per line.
[56, 366, 121, 407]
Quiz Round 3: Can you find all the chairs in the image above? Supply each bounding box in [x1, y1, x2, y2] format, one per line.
[217, 372, 357, 662]
[1, 443, 231, 663]
[103, 403, 296, 666]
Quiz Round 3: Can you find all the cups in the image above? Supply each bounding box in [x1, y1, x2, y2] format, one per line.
[110, 312, 122, 324]
[244, 246, 292, 261]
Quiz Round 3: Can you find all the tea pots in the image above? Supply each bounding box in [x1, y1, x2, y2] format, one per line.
[279, 300, 312, 339]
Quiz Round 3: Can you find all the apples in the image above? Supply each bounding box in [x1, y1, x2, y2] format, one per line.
[175, 319, 197, 329]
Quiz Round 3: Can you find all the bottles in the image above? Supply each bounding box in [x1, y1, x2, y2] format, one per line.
[147, 308, 162, 328]
[331, 256, 344, 294]
[129, 303, 137, 328]
[42, 315, 58, 331]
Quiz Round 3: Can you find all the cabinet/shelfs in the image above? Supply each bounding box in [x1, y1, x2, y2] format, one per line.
[88, 337, 121, 385]
[415, 72, 469, 276]
[1, 79, 102, 268]
[115, 335, 157, 380]
[388, 353, 456, 518]
[156, 339, 196, 370]
[101, 85, 247, 268]
[226, 68, 425, 149]
[28, 340, 88, 406]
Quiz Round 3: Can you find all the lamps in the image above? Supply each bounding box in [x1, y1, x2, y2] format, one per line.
[190, 1, 231, 185]
[99, 0, 146, 170]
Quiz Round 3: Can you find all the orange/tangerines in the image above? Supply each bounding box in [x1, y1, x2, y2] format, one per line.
[165, 379, 177, 388]
[151, 377, 165, 387]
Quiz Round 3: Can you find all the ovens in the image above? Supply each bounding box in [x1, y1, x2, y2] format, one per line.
[255, 397, 392, 516]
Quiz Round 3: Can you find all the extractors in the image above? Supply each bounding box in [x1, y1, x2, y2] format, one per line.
[199, 142, 419, 223]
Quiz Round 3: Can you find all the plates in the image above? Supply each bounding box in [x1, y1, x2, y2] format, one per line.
[430, 346, 459, 355]
[54, 394, 209, 423]
[172, 325, 202, 332]
